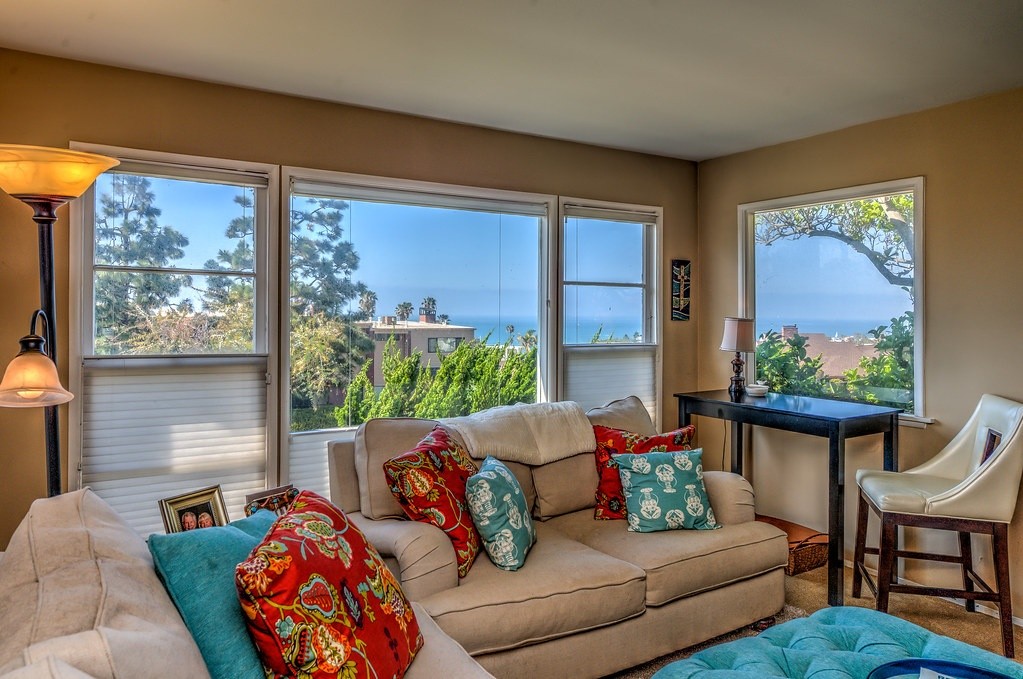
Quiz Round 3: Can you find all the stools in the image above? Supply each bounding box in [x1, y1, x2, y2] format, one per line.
[647, 605, 1023, 679]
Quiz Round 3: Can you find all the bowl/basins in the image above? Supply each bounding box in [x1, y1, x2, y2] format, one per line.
[745, 385, 769, 396]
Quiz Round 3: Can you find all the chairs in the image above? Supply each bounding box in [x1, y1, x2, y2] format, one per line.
[852, 394, 1023, 658]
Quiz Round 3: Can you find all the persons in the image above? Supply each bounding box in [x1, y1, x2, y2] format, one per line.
[181, 511, 214, 531]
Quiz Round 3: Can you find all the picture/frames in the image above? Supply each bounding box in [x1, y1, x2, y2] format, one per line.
[157, 484, 230, 534]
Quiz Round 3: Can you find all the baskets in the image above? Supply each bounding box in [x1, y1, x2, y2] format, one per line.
[784, 532, 829, 576]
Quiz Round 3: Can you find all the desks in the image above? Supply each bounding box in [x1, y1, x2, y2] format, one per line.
[672, 389, 905, 607]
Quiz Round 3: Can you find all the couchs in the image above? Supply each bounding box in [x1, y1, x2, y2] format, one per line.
[0, 486, 497, 679]
[326, 395, 789, 679]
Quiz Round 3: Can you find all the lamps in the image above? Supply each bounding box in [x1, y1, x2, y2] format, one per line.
[719, 316, 755, 391]
[0, 144, 121, 498]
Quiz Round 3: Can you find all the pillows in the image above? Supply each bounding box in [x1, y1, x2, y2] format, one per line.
[611, 447, 723, 532]
[235, 490, 425, 679]
[148, 508, 280, 679]
[464, 453, 537, 571]
[383, 422, 483, 578]
[594, 424, 697, 520]
